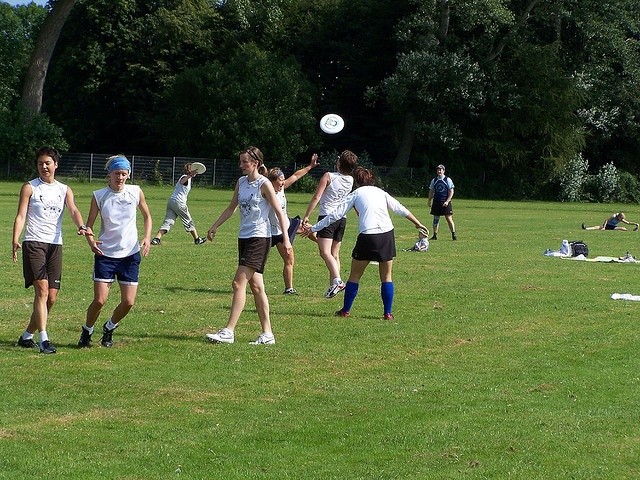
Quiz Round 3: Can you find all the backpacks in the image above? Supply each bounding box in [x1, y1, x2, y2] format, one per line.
[570, 240, 588, 257]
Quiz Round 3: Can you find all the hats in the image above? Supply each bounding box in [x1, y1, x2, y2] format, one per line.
[436, 164, 445, 170]
[419, 228, 429, 237]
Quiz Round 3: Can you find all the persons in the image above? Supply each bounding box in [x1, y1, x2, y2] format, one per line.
[428, 166, 458, 241]
[151, 161, 207, 247]
[301, 149, 357, 298]
[78, 154, 153, 349]
[579, 211, 639, 232]
[411, 226, 428, 252]
[206, 145, 293, 347]
[300, 167, 429, 320]
[259, 153, 319, 297]
[12, 148, 94, 352]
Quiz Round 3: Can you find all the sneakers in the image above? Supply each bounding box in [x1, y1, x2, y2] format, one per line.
[384, 313, 394, 320]
[205, 329, 234, 344]
[249, 334, 275, 344]
[195, 236, 207, 244]
[40, 340, 56, 353]
[335, 310, 350, 318]
[283, 287, 296, 295]
[18, 336, 38, 348]
[78, 326, 94, 347]
[98, 321, 119, 348]
[430, 236, 437, 239]
[151, 239, 161, 245]
[452, 235, 456, 240]
[325, 280, 346, 298]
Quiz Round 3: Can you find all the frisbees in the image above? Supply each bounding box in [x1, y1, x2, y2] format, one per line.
[191, 162, 206, 174]
[320, 114, 345, 134]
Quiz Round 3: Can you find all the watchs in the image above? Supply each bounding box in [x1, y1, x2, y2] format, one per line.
[79, 225, 86, 231]
